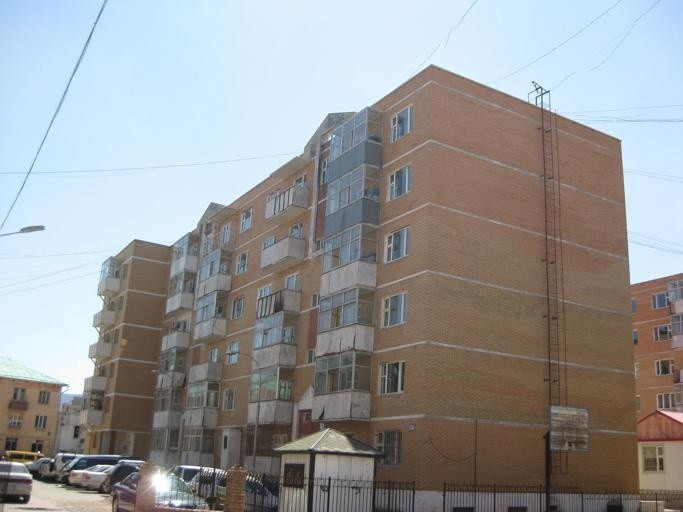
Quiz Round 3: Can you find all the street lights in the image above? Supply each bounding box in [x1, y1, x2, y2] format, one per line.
[152, 368, 176, 466]
[225, 350, 262, 474]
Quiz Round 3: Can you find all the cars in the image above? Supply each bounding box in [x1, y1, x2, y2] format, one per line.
[110, 470, 209, 512]
[172, 465, 210, 483]
[25, 452, 150, 494]
[189, 469, 278, 511]
[0, 459, 33, 503]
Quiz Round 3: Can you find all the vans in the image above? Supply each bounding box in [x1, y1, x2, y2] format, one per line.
[4, 450, 45, 463]
